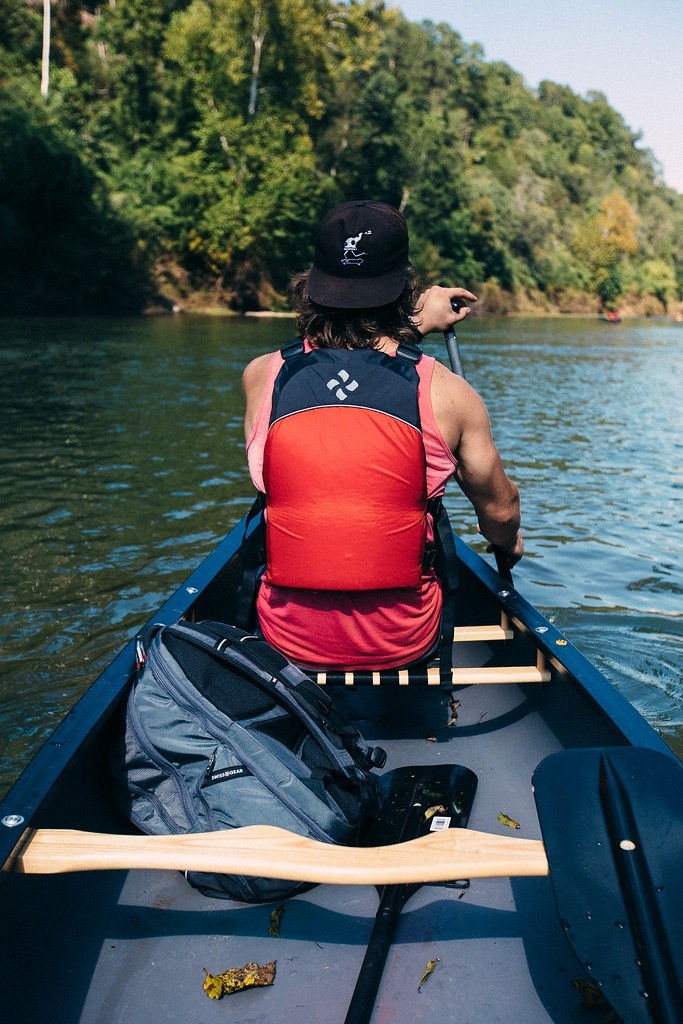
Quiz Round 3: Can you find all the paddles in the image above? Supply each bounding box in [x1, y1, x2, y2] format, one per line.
[344, 763, 479, 1024]
[443, 298, 517, 586]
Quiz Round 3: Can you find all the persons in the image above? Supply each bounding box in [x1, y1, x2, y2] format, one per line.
[238, 199, 519, 677]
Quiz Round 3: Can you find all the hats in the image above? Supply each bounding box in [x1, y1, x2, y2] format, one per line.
[307, 200, 410, 308]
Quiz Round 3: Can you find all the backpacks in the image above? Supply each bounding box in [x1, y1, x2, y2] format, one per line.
[108, 619, 388, 904]
